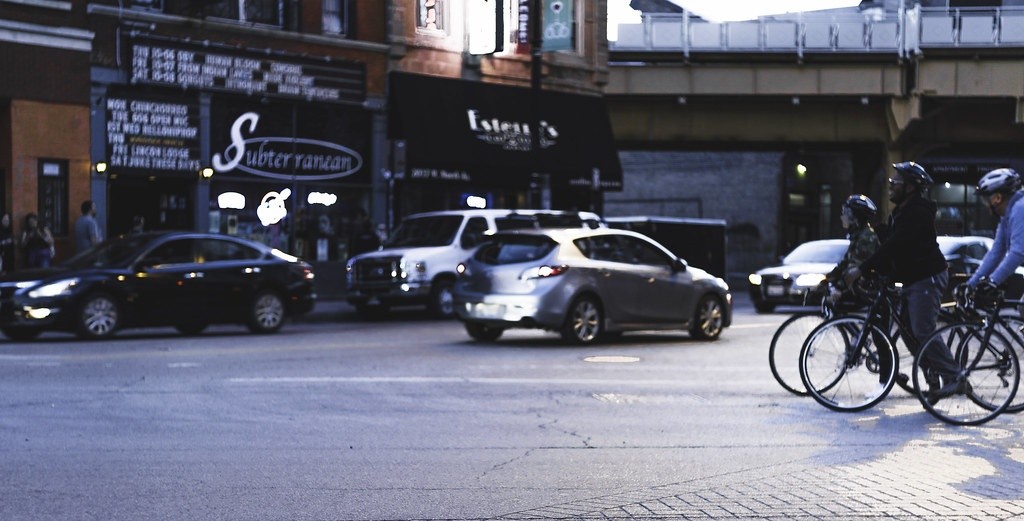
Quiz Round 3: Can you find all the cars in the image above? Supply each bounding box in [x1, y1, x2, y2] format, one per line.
[747, 238, 853, 318]
[452, 223, 734, 348]
[935, 235, 1024, 308]
[0, 228, 319, 344]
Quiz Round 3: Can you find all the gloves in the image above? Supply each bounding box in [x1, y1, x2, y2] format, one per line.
[953, 283, 973, 309]
[973, 279, 999, 305]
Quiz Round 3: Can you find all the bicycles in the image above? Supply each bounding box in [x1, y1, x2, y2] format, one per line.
[768, 268, 1024, 426]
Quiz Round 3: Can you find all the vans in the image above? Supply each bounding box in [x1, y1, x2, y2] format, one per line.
[344, 207, 616, 320]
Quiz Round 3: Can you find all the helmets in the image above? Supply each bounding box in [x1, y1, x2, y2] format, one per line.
[846, 194, 877, 219]
[893, 161, 934, 193]
[973, 167, 1021, 194]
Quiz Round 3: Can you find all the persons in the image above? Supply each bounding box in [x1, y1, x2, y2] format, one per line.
[959, 167, 1024, 334]
[819, 196, 894, 388]
[131, 215, 144, 234]
[18, 213, 55, 268]
[73, 199, 104, 260]
[0, 211, 12, 279]
[842, 158, 972, 405]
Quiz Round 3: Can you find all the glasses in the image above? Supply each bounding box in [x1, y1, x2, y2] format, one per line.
[888, 177, 907, 185]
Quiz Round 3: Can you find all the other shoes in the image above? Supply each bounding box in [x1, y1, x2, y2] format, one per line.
[927, 378, 940, 406]
[934, 378, 972, 398]
